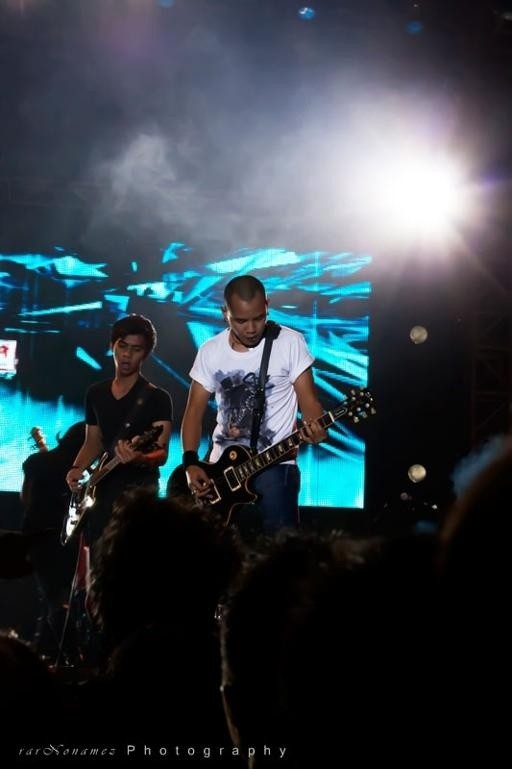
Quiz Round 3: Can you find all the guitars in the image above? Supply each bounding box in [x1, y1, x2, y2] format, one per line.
[61, 423, 167, 544]
[165, 390, 378, 526]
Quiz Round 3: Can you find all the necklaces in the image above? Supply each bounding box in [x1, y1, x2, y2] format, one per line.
[234, 334, 257, 351]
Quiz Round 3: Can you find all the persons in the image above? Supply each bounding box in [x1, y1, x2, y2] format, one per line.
[0, 438, 512, 768]
[66, 313, 172, 543]
[180, 274, 329, 538]
